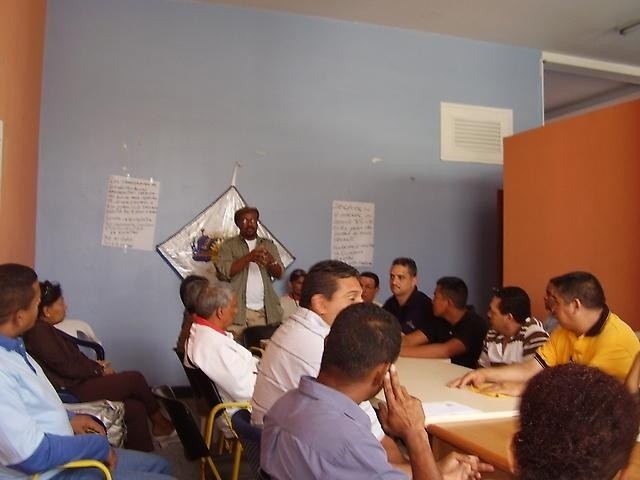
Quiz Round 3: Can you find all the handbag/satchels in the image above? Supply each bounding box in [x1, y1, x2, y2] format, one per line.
[64, 399, 126, 447]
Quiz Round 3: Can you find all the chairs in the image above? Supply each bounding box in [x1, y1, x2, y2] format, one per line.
[147, 345, 272, 479]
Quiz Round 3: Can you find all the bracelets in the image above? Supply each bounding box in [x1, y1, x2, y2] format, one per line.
[268, 261, 279, 266]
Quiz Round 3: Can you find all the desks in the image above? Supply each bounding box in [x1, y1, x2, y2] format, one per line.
[372, 357, 639, 479]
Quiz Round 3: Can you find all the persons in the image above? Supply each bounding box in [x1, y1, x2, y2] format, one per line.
[539, 278, 561, 335]
[277, 270, 308, 321]
[0, 263, 175, 480]
[504, 361, 640, 480]
[474, 284, 551, 369]
[182, 280, 262, 417]
[358, 270, 384, 308]
[380, 256, 434, 335]
[249, 259, 412, 466]
[259, 301, 497, 480]
[176, 273, 209, 355]
[446, 270, 640, 400]
[397, 275, 488, 369]
[17, 279, 179, 454]
[214, 205, 288, 344]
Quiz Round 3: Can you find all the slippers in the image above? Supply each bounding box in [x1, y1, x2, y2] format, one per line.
[154, 425, 178, 440]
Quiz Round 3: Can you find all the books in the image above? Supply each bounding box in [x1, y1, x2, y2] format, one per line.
[419, 399, 521, 428]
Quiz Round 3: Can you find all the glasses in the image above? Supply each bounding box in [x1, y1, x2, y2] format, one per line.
[43, 280, 53, 299]
[239, 217, 257, 225]
